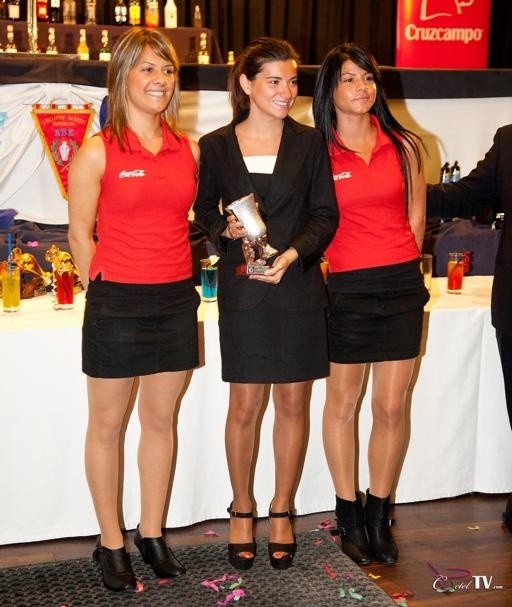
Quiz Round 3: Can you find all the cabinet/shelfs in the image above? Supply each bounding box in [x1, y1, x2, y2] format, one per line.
[0, 0, 216, 67]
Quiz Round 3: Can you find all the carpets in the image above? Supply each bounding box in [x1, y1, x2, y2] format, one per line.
[0, 528, 394, 603]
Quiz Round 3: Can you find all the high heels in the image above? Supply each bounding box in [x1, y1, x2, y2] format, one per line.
[364, 489, 399, 565]
[227, 501, 256, 570]
[135, 525, 186, 579]
[334, 494, 372, 565]
[93, 537, 136, 592]
[267, 494, 298, 569]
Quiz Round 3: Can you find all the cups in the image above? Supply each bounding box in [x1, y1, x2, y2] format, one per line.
[1, 266, 21, 313]
[446, 252, 466, 294]
[200, 258, 220, 305]
[52, 260, 75, 309]
[418, 255, 433, 290]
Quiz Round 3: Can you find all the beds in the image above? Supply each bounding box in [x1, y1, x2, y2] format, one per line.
[0, 272, 511, 536]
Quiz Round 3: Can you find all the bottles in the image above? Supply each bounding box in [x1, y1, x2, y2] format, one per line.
[114, 0, 129, 24]
[36, 0, 49, 22]
[144, 0, 159, 28]
[62, 0, 77, 26]
[49, 0, 62, 23]
[86, 0, 97, 25]
[128, 0, 140, 27]
[7, 0, 21, 21]
[163, 0, 177, 29]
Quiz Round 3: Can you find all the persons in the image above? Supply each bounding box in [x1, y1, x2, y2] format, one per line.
[314, 43, 427, 566]
[68, 26, 200, 593]
[194, 38, 339, 570]
[426, 124, 511, 531]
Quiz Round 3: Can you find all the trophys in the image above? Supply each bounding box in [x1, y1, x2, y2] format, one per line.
[225, 193, 278, 276]
